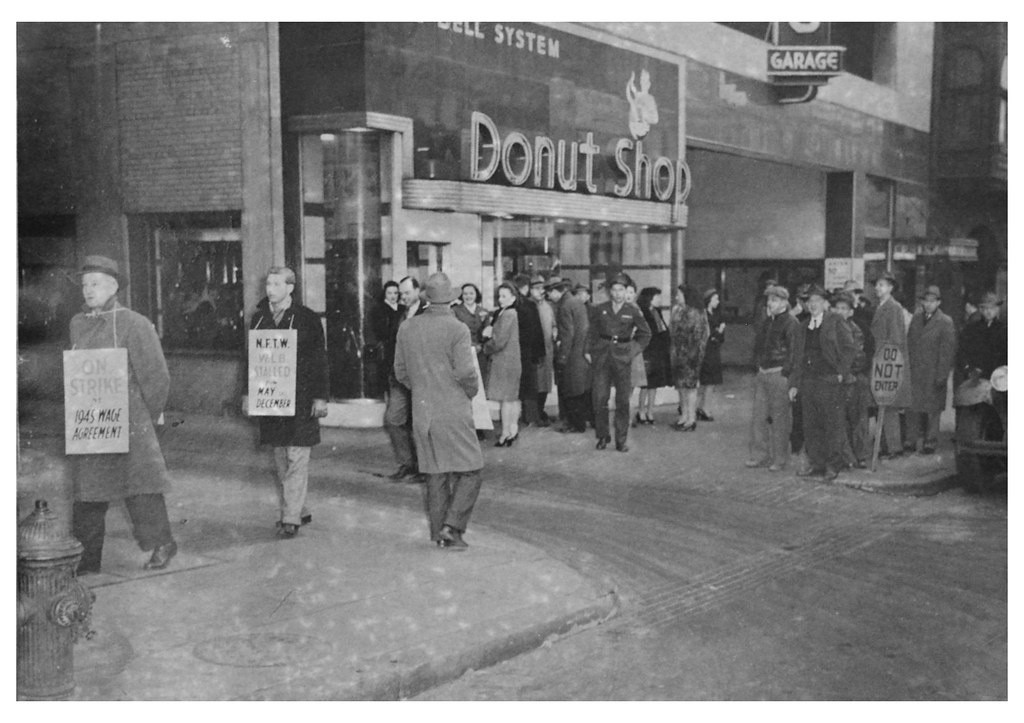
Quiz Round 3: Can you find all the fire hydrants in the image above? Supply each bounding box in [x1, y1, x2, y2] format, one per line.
[16, 499, 97, 701]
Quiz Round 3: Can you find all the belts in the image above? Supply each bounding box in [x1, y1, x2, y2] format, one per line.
[600, 335, 630, 344]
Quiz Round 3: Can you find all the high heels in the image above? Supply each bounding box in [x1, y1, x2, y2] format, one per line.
[636, 412, 649, 425]
[672, 420, 697, 431]
[645, 413, 655, 424]
[696, 409, 714, 421]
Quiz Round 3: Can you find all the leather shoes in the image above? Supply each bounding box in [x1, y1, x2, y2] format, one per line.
[143, 541, 178, 571]
[410, 473, 428, 483]
[276, 524, 299, 537]
[388, 465, 419, 479]
[276, 515, 311, 526]
[439, 525, 469, 548]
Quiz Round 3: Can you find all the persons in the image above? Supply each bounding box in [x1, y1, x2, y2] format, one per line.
[955, 290, 1009, 441]
[742, 275, 955, 483]
[68, 254, 179, 577]
[510, 269, 727, 454]
[372, 274, 523, 484]
[393, 271, 485, 549]
[239, 265, 328, 539]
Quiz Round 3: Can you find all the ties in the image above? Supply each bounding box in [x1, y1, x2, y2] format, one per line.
[813, 319, 817, 330]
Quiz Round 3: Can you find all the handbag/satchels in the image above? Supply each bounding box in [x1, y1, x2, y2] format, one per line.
[954, 377, 993, 406]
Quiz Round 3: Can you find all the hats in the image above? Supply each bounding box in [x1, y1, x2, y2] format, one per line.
[418, 272, 573, 303]
[609, 272, 631, 288]
[762, 272, 1005, 305]
[704, 289, 717, 300]
[75, 255, 130, 292]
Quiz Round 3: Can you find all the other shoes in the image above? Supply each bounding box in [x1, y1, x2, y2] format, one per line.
[796, 466, 817, 476]
[769, 464, 784, 470]
[823, 470, 839, 480]
[494, 434, 518, 447]
[841, 441, 937, 469]
[596, 435, 611, 449]
[616, 443, 629, 451]
[527, 415, 590, 433]
[745, 459, 761, 467]
